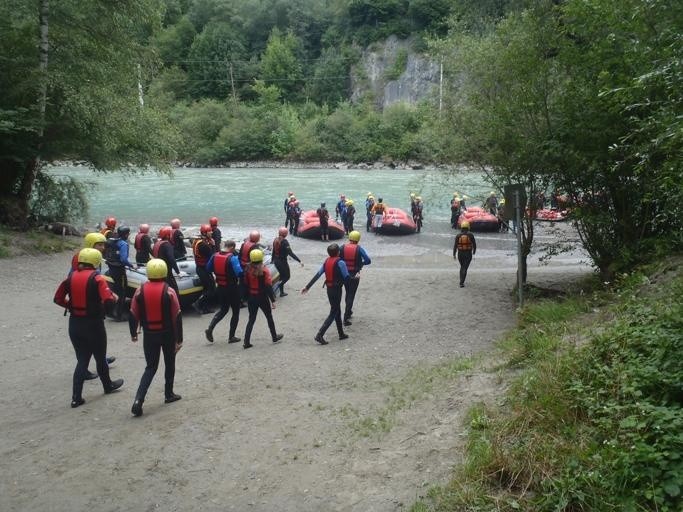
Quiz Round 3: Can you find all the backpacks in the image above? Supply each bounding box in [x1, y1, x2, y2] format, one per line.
[105, 238, 126, 267]
[320, 216, 328, 228]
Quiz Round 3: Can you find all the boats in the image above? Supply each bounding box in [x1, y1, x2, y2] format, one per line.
[524, 205, 569, 225]
[457, 207, 500, 233]
[556, 191, 603, 214]
[376, 207, 416, 237]
[97, 253, 284, 309]
[298, 207, 345, 241]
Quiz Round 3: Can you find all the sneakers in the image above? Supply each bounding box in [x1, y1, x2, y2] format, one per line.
[322, 239, 329, 242]
[105, 356, 115, 364]
[83, 369, 99, 380]
[459, 281, 465, 288]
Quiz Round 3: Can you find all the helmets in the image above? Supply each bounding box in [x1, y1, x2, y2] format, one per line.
[250, 249, 264, 262]
[349, 230, 361, 242]
[78, 248, 103, 268]
[250, 230, 259, 241]
[278, 227, 289, 237]
[106, 215, 219, 239]
[83, 231, 106, 248]
[490, 190, 506, 204]
[340, 192, 384, 205]
[410, 192, 422, 209]
[452, 192, 467, 201]
[146, 258, 168, 280]
[287, 192, 299, 207]
[460, 221, 471, 231]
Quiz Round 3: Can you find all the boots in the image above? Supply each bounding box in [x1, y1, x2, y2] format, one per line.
[100, 369, 125, 394]
[227, 320, 241, 342]
[279, 280, 288, 297]
[414, 218, 422, 233]
[205, 317, 220, 342]
[131, 388, 146, 415]
[270, 325, 284, 342]
[366, 223, 385, 236]
[313, 319, 331, 345]
[243, 330, 254, 349]
[335, 321, 349, 340]
[284, 223, 298, 237]
[343, 306, 354, 327]
[104, 292, 130, 322]
[71, 381, 85, 408]
[192, 294, 215, 314]
[164, 376, 182, 403]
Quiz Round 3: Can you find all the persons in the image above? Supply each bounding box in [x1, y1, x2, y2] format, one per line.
[269, 226, 304, 298]
[205, 230, 284, 349]
[537, 187, 561, 211]
[335, 191, 424, 235]
[450, 190, 509, 233]
[129, 258, 183, 418]
[339, 231, 371, 326]
[317, 202, 329, 242]
[300, 243, 351, 345]
[453, 221, 476, 287]
[53, 233, 123, 408]
[101, 217, 221, 323]
[284, 192, 302, 237]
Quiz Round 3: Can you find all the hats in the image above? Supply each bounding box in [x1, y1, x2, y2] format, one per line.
[320, 202, 326, 206]
